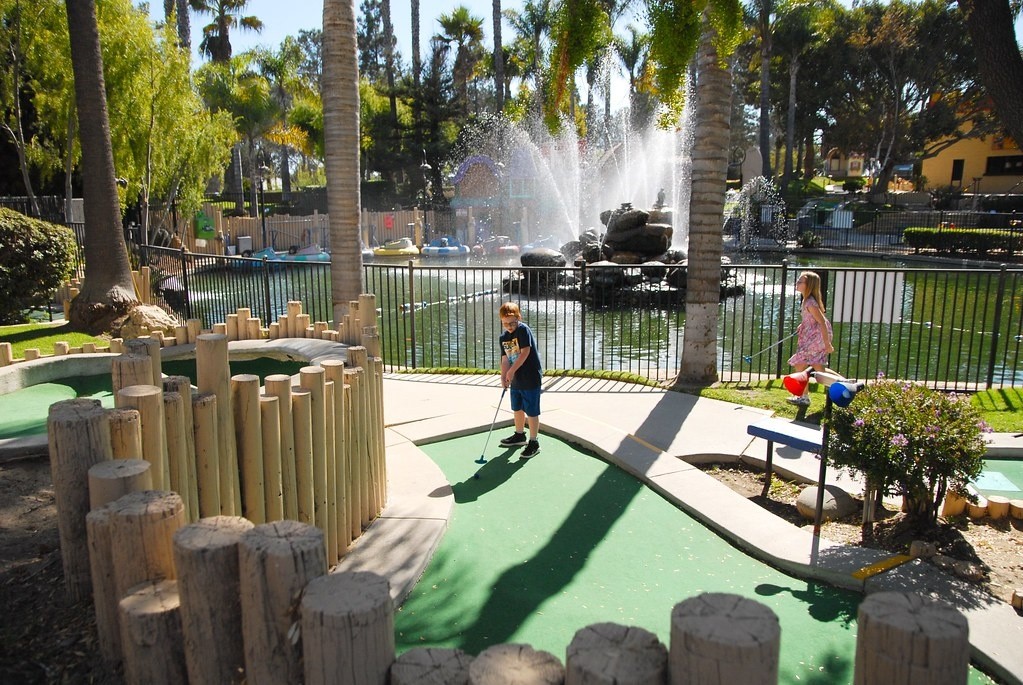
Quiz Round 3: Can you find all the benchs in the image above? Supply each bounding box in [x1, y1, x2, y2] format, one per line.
[748, 421, 865, 502]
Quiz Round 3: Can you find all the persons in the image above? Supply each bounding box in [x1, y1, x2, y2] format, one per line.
[786, 270, 856, 406]
[499, 303, 544, 457]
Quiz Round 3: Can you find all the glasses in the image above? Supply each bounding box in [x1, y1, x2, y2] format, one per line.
[502, 319, 518, 327]
[797, 280, 806, 284]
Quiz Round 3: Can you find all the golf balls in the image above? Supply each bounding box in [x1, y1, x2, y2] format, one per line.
[474, 473, 480, 479]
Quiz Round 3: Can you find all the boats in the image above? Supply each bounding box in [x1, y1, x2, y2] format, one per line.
[248, 243, 332, 268]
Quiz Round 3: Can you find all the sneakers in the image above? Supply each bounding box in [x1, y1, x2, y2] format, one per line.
[521, 440, 541, 458]
[787, 394, 811, 405]
[500, 432, 527, 446]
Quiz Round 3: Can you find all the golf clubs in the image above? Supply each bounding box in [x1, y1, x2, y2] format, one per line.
[742, 331, 798, 364]
[474, 380, 509, 464]
